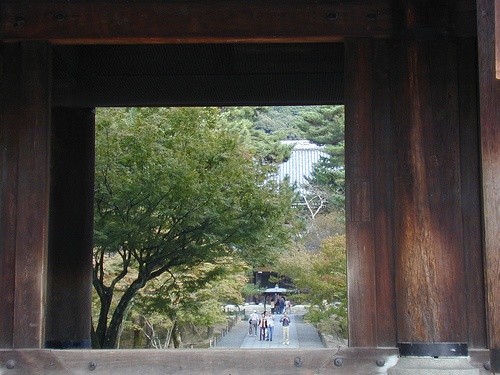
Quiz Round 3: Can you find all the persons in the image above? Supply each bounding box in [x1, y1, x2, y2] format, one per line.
[258, 311, 275, 341]
[280, 312, 290, 345]
[271, 297, 285, 314]
[249, 310, 259, 336]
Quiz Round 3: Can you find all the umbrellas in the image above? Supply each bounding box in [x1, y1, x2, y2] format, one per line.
[262, 285, 291, 292]
